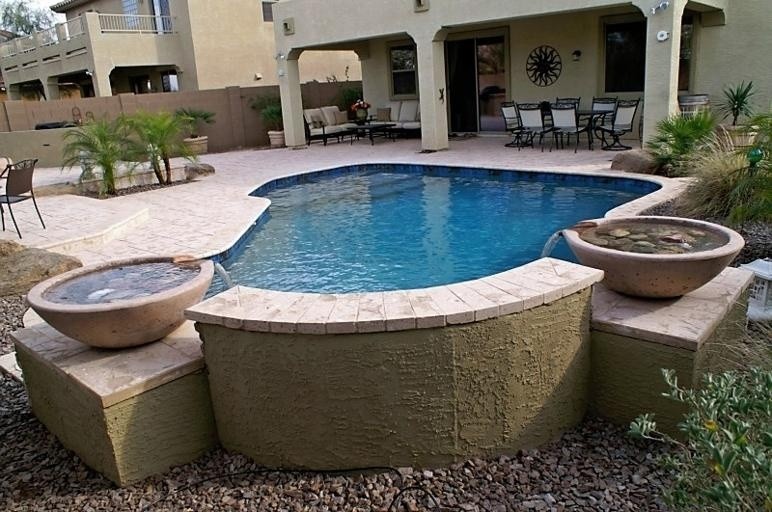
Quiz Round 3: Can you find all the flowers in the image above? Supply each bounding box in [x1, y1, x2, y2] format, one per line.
[351, 99, 370, 111]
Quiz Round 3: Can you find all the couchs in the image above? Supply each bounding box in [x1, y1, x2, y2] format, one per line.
[368, 101, 421, 139]
[303, 106, 358, 146]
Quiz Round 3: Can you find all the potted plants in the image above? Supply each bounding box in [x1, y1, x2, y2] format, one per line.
[713, 79, 762, 150]
[173, 107, 216, 155]
[262, 105, 285, 148]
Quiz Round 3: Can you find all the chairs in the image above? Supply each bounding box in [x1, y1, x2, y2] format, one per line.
[0, 158, 46, 239]
[500, 96, 641, 152]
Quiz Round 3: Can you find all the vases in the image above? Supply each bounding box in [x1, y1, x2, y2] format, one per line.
[356, 108, 366, 123]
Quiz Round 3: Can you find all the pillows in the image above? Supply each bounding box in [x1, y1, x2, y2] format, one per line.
[334, 111, 355, 125]
[376, 107, 391, 122]
[311, 114, 326, 128]
